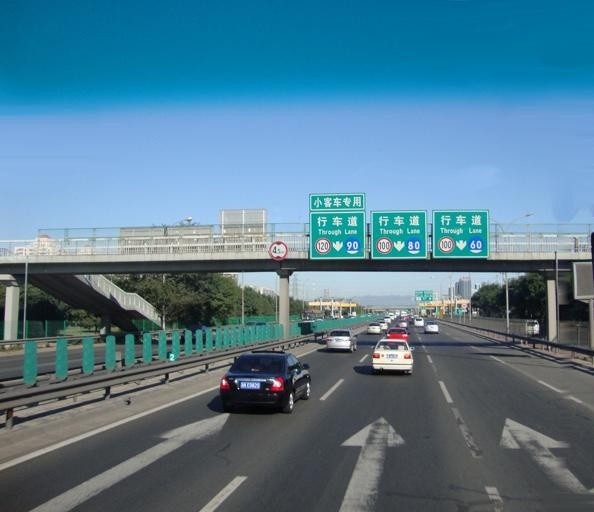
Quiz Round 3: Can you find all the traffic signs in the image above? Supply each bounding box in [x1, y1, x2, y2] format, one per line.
[371, 210, 428, 261]
[424, 290, 434, 301]
[415, 291, 424, 302]
[431, 209, 490, 260]
[307, 193, 367, 261]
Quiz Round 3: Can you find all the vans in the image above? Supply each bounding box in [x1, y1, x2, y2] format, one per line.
[518, 318, 541, 336]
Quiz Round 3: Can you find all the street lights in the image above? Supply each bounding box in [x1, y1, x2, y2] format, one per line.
[487, 210, 537, 334]
[161, 216, 193, 331]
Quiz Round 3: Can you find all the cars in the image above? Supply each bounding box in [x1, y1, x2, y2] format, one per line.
[218, 348, 313, 415]
[370, 338, 416, 376]
[324, 328, 358, 354]
[180, 322, 210, 344]
[243, 307, 480, 339]
